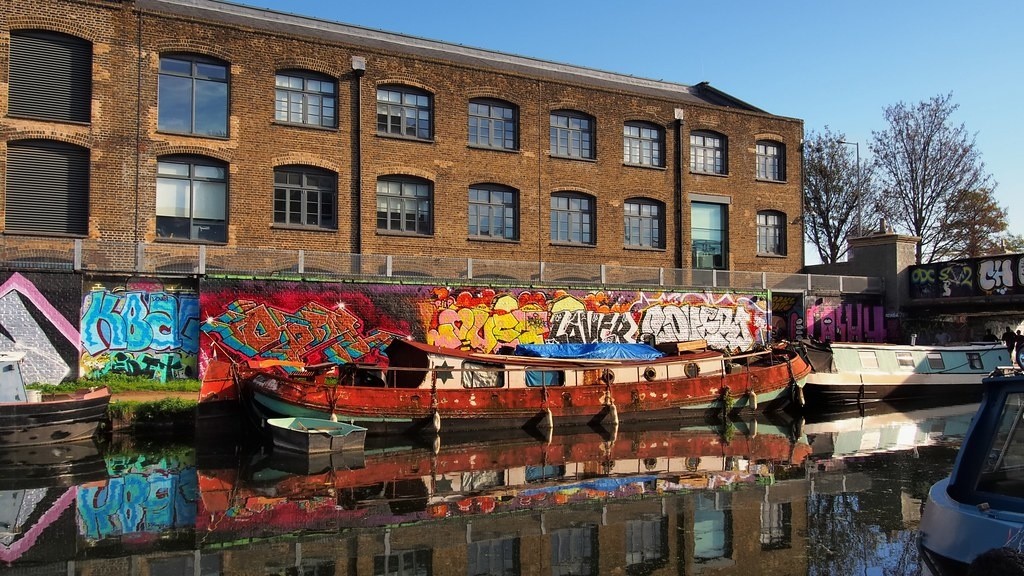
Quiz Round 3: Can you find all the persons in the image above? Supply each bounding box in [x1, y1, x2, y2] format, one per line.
[983, 327, 1024, 363]
[935, 325, 952, 345]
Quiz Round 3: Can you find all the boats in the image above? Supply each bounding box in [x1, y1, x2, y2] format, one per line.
[0, 437, 111, 491]
[213, 333, 812, 439]
[250, 420, 814, 503]
[915, 366, 1024, 576]
[796, 341, 1023, 409]
[265, 416, 368, 456]
[0, 386, 115, 448]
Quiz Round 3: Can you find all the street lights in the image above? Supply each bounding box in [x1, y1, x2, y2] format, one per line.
[836, 138, 863, 236]
[801, 140, 813, 275]
